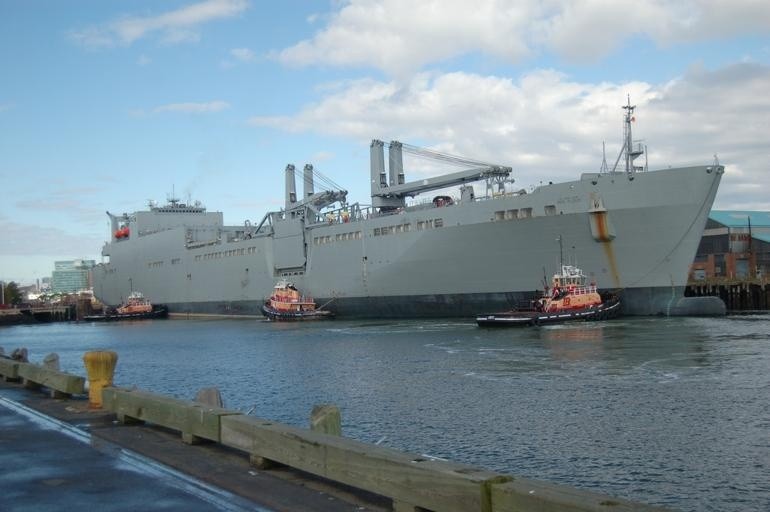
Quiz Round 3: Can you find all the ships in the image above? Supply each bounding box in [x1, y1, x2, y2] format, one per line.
[90, 95, 729, 318]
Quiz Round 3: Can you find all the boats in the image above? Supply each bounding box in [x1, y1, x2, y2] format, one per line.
[83, 280, 167, 322]
[261, 279, 335, 320]
[475, 235, 622, 331]
